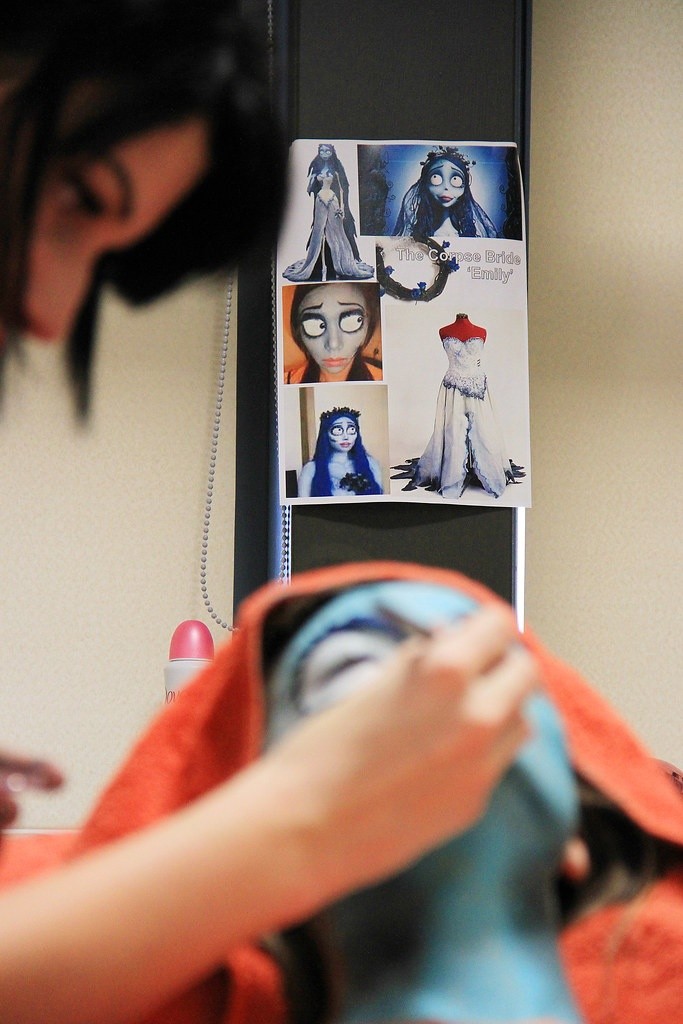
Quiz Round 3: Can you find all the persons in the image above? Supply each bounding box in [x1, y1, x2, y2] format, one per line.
[419, 312, 507, 500]
[233, 562, 592, 1024]
[0, 0, 534, 1024]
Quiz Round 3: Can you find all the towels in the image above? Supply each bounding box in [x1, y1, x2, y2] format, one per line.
[0, 562, 681, 1024]
